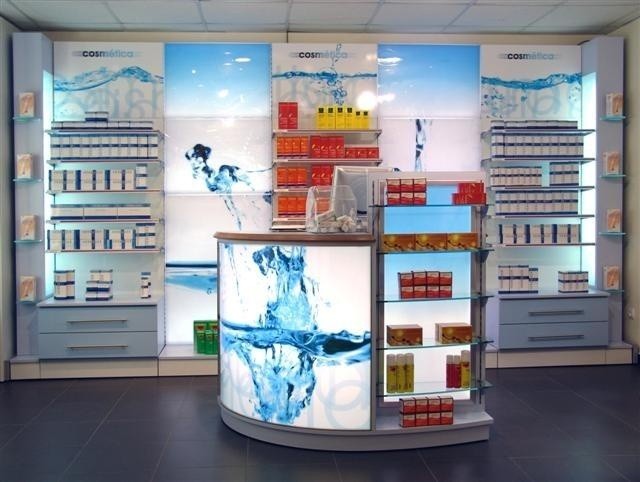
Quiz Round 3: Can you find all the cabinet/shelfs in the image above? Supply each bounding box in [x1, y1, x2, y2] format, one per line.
[7, 113, 45, 307]
[39, 126, 171, 253]
[483, 282, 612, 353]
[482, 125, 600, 250]
[366, 200, 499, 453]
[597, 113, 629, 298]
[271, 125, 383, 232]
[33, 292, 166, 363]
